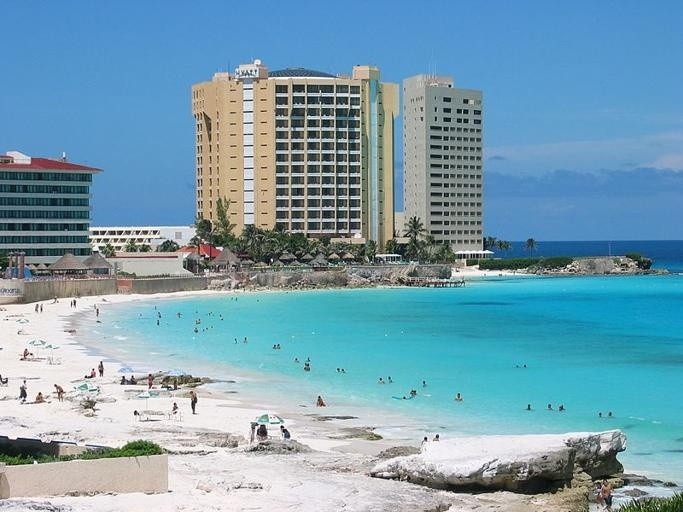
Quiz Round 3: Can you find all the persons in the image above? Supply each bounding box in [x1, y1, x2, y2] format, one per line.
[18, 348, 104, 404]
[120, 374, 196, 415]
[148, 304, 224, 333]
[258, 424, 290, 441]
[378, 376, 615, 443]
[234, 336, 347, 408]
[35, 296, 100, 317]
[594, 482, 604, 510]
[601, 479, 616, 512]
